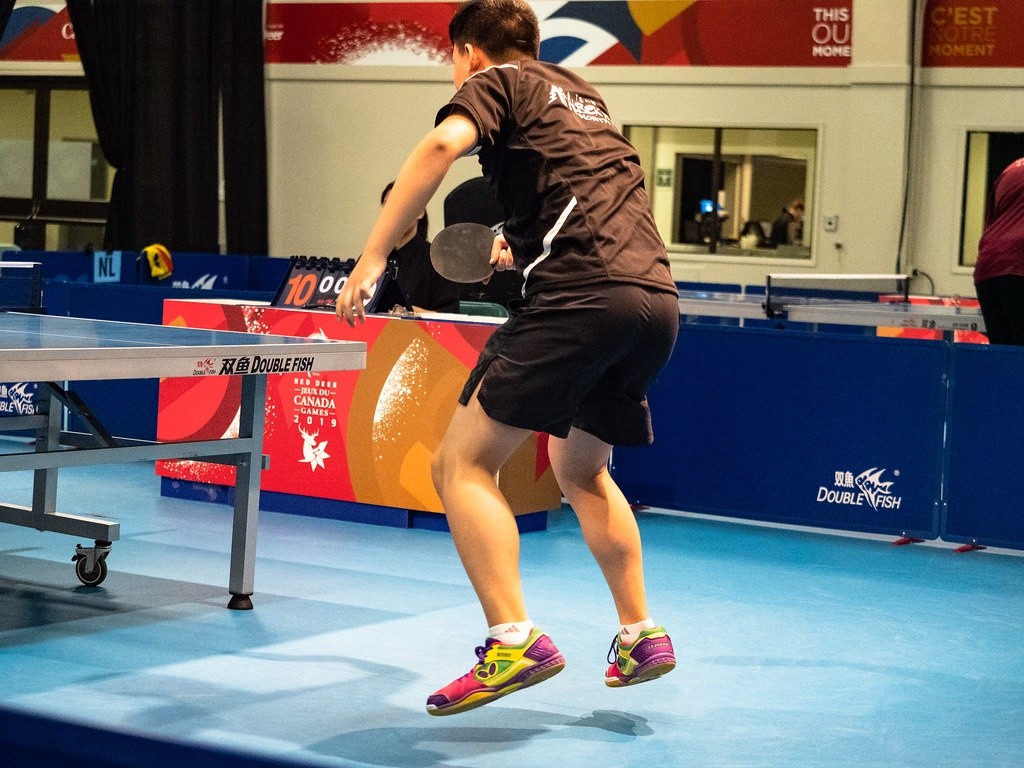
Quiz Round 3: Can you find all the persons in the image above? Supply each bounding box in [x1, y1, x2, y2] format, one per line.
[771, 197, 804, 250]
[970, 158, 1024, 345]
[335, 0, 680, 717]
[740, 221, 770, 250]
[364, 181, 460, 315]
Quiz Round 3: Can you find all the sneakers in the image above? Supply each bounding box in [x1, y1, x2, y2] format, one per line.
[426, 624, 566, 717]
[604, 625, 676, 687]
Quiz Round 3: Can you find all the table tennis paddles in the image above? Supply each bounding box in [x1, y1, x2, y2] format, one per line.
[426, 222, 498, 286]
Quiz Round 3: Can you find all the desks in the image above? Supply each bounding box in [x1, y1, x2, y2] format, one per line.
[677, 289, 986, 342]
[1, 311, 367, 613]
[876, 293, 990, 345]
[156, 299, 562, 534]
